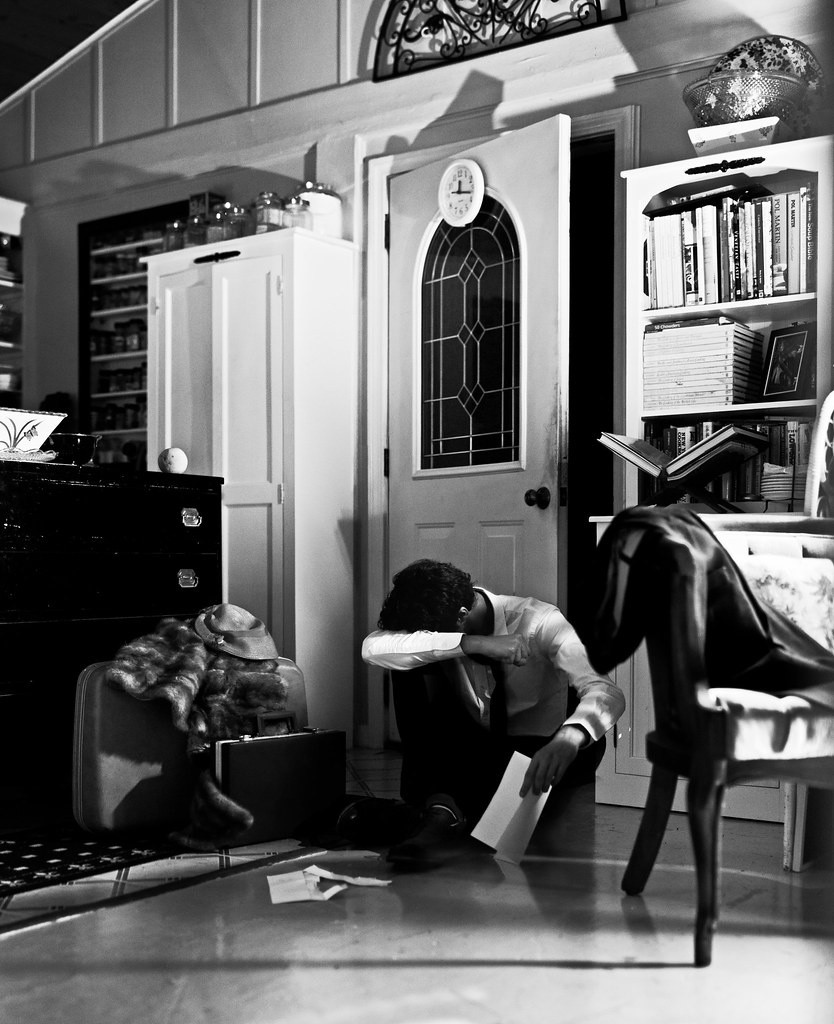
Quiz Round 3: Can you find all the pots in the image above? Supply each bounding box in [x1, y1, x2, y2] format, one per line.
[47, 432, 102, 465]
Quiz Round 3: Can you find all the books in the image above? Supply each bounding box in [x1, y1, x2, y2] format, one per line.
[596, 183, 817, 506]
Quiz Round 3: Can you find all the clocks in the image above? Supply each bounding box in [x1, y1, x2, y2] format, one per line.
[437, 158, 485, 227]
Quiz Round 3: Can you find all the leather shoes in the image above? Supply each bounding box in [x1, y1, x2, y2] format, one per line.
[336, 798, 408, 843]
[387, 822, 471, 863]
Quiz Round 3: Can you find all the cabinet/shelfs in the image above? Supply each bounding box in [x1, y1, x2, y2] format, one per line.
[77, 199, 190, 470]
[589, 131, 834, 824]
[1, 197, 42, 411]
[139, 227, 359, 751]
[0, 459, 227, 827]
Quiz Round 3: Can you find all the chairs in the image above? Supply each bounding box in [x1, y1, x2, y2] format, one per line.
[620, 534, 833, 967]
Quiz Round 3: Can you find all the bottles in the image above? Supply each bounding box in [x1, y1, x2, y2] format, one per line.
[84, 237, 146, 429]
[163, 184, 346, 247]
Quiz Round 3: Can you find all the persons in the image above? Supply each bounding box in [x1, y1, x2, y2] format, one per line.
[360, 557, 625, 863]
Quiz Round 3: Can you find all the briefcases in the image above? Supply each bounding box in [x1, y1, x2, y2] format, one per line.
[215, 709, 348, 841]
[72, 656, 307, 832]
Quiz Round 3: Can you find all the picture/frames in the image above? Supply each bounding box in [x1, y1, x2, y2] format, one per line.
[756, 321, 816, 403]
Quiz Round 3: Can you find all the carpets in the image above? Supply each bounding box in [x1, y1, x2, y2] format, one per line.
[1, 823, 329, 934]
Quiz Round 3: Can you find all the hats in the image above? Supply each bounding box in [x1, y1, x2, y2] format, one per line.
[194, 603, 280, 660]
